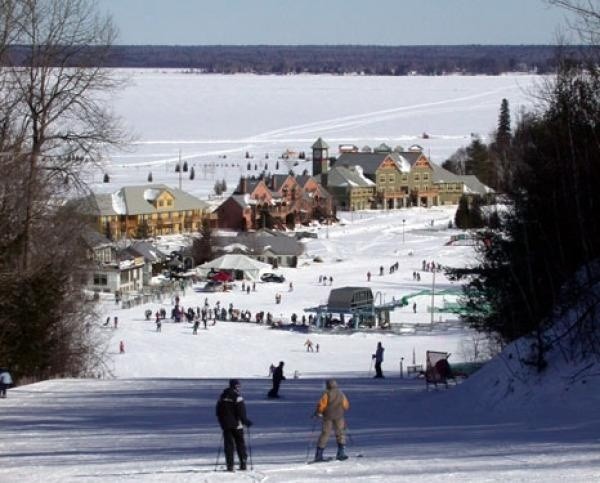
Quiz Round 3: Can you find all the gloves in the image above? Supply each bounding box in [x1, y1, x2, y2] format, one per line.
[245, 419, 252, 427]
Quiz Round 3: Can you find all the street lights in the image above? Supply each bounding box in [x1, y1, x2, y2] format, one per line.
[402, 218, 406, 241]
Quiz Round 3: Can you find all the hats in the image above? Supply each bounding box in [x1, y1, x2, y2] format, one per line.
[229, 379, 240, 389]
[326, 380, 336, 389]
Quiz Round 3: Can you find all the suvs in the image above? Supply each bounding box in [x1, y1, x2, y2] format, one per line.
[261, 272, 285, 283]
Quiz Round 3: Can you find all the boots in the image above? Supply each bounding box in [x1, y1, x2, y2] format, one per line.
[316, 446, 324, 460]
[337, 443, 348, 459]
[226, 455, 233, 470]
[240, 455, 247, 469]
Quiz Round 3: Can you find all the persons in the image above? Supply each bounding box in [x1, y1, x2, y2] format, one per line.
[304, 338, 314, 351]
[371, 341, 385, 378]
[0, 366, 13, 398]
[268, 362, 274, 376]
[267, 360, 285, 398]
[215, 378, 253, 471]
[140, 274, 357, 336]
[114, 316, 118, 327]
[366, 257, 442, 283]
[119, 339, 126, 354]
[104, 316, 110, 325]
[311, 378, 351, 460]
